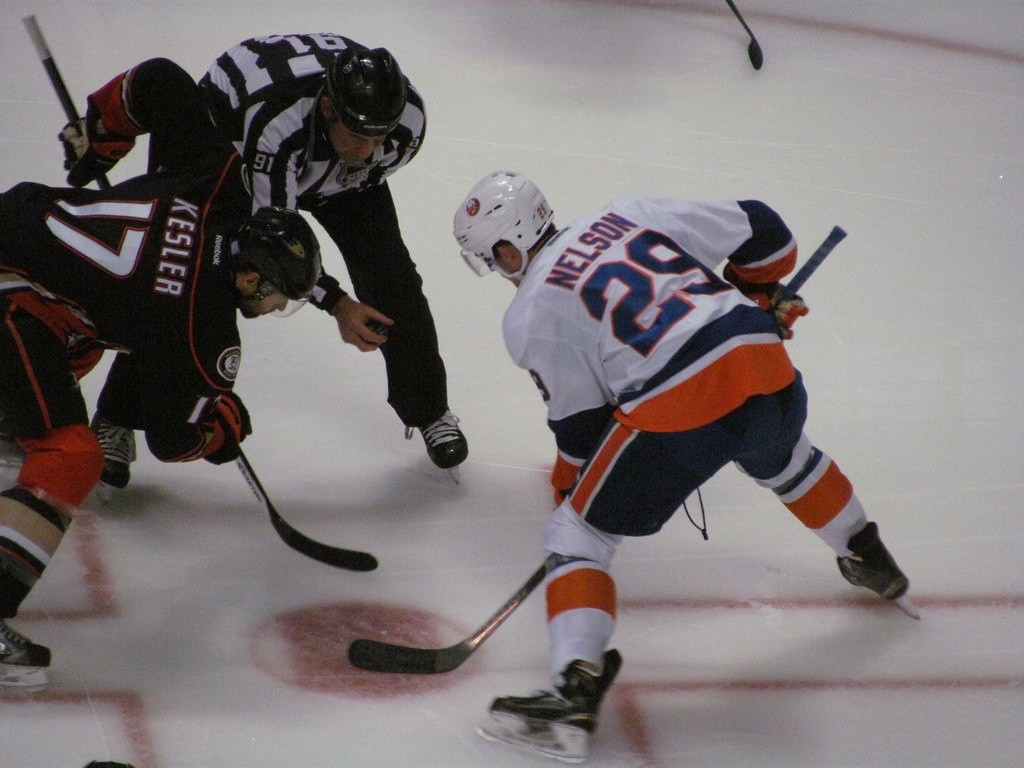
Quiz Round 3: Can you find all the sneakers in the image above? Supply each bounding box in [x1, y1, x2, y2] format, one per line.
[836, 521, 923, 618]
[405, 405, 468, 485]
[89, 410, 137, 503]
[475, 649, 622, 764]
[0, 619, 51, 692]
[0, 417, 26, 468]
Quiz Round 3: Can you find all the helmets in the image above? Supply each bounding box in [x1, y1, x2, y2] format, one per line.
[453, 170, 555, 277]
[326, 45, 407, 140]
[220, 206, 322, 302]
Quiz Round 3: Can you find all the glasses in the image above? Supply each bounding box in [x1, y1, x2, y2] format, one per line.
[340, 125, 387, 150]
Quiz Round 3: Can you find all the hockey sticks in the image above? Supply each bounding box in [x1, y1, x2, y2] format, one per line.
[725, 0, 763, 71]
[343, 225, 848, 673]
[21, 10, 384, 573]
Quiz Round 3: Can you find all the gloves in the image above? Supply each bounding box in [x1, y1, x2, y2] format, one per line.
[744, 281, 809, 340]
[57, 117, 135, 188]
[550, 450, 588, 506]
[193, 390, 252, 465]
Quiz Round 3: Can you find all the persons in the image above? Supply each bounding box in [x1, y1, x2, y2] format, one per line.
[0, 54, 321, 693]
[88, 34, 469, 504]
[452, 168, 919, 765]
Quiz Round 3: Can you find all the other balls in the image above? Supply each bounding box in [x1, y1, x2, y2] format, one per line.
[366, 321, 387, 344]
